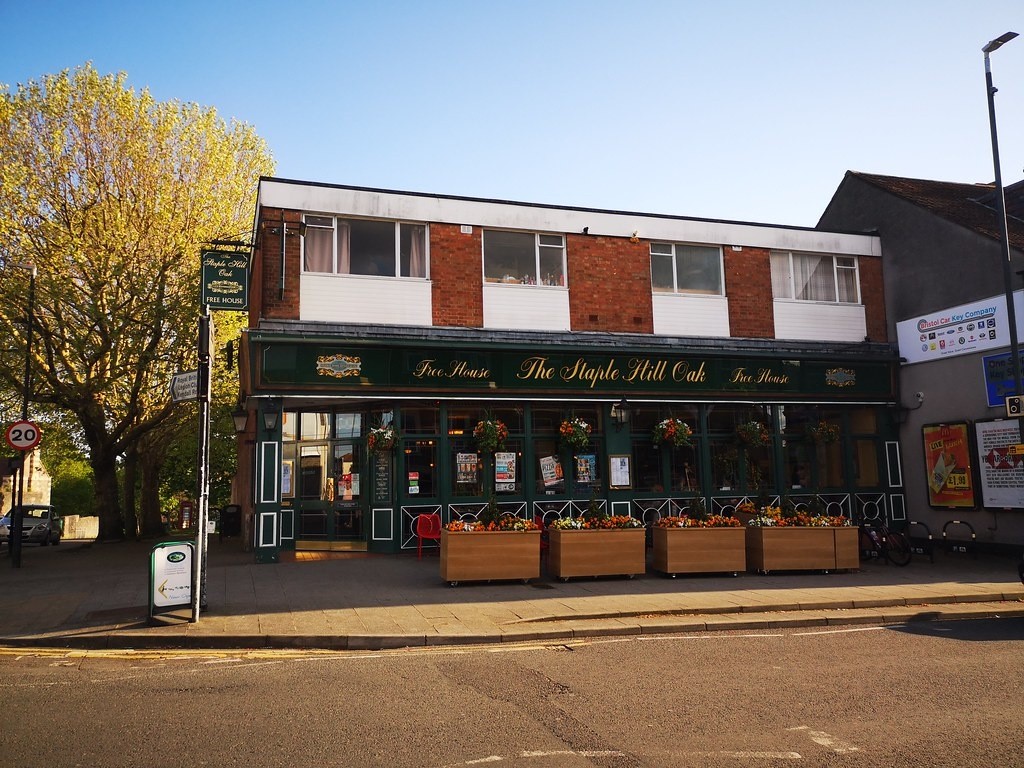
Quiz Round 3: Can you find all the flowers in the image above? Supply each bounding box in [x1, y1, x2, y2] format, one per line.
[653, 417, 693, 449]
[735, 420, 770, 447]
[811, 421, 841, 444]
[656, 495, 740, 527]
[446, 495, 538, 530]
[473, 420, 508, 455]
[746, 506, 853, 527]
[559, 418, 592, 451]
[366, 427, 400, 452]
[548, 492, 643, 529]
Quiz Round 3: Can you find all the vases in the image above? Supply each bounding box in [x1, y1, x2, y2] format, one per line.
[439, 527, 542, 582]
[380, 431, 395, 449]
[550, 528, 646, 578]
[653, 526, 747, 573]
[757, 527, 860, 572]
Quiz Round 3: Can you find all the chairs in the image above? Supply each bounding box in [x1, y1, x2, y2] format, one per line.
[417, 515, 442, 559]
[41, 511, 48, 519]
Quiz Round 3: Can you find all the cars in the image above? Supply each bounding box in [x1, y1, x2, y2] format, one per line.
[0, 505, 63, 547]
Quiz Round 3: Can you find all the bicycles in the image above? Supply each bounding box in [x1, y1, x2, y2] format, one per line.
[860, 514, 912, 567]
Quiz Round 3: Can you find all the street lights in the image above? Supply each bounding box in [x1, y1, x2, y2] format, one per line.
[983, 30, 1024, 446]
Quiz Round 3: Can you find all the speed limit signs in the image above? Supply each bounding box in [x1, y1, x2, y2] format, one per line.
[6, 421, 40, 451]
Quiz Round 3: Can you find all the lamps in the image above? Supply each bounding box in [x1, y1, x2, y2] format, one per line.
[262, 395, 280, 440]
[232, 404, 257, 434]
[611, 394, 632, 434]
